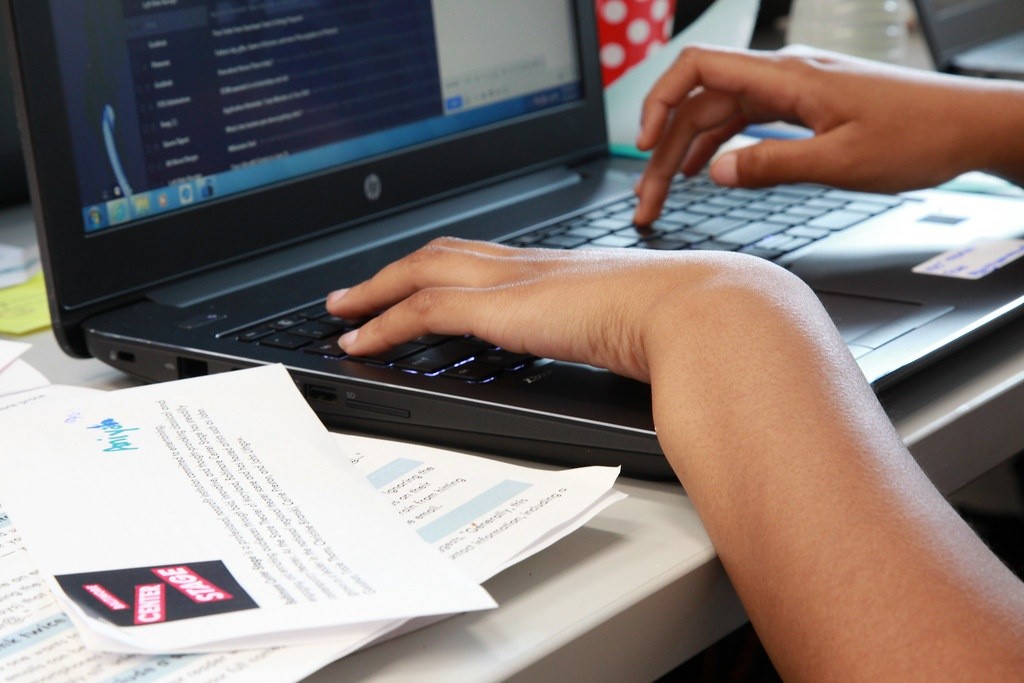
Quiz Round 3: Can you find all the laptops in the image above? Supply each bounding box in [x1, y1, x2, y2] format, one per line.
[0, 1, 1023, 486]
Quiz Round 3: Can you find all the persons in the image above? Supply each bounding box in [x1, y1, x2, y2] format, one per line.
[328, 44, 1024, 683]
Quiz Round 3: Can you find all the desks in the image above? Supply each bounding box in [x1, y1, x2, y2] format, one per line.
[0, 201, 1024, 683]
